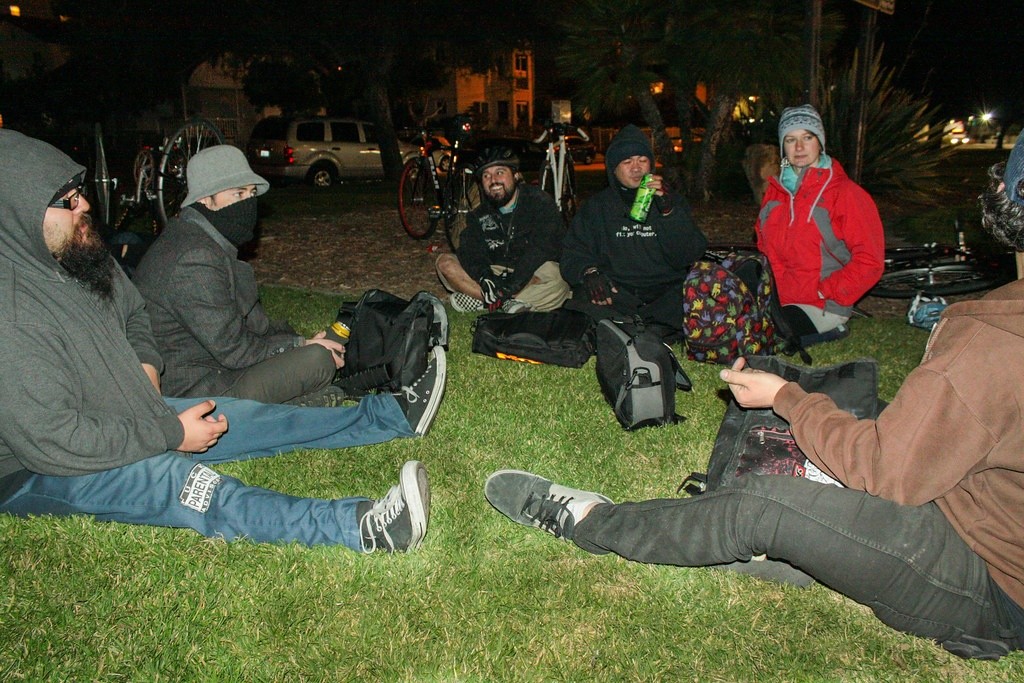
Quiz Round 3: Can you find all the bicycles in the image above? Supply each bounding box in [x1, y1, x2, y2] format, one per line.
[90, 117, 224, 242]
[863, 240, 1015, 299]
[528, 121, 591, 228]
[397, 117, 489, 254]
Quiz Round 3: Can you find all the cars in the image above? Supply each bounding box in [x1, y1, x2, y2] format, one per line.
[547, 136, 597, 165]
[669, 135, 702, 154]
[949, 132, 970, 145]
[246, 113, 424, 195]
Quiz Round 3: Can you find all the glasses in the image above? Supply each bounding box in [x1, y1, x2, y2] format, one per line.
[47, 185, 88, 210]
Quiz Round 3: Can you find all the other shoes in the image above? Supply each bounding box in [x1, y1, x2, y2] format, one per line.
[281, 385, 344, 408]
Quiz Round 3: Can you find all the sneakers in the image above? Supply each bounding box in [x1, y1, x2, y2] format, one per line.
[356, 461, 430, 557]
[500, 298, 532, 314]
[399, 346, 448, 437]
[484, 469, 615, 541]
[722, 554, 816, 592]
[450, 291, 485, 312]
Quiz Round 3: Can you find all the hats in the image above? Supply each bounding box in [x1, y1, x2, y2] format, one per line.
[47, 174, 81, 207]
[178, 144, 271, 208]
[608, 141, 652, 170]
[778, 103, 832, 226]
[1003, 127, 1024, 206]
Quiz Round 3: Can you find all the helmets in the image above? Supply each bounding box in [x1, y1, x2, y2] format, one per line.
[473, 148, 519, 181]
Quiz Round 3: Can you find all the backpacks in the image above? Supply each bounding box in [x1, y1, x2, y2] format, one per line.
[683, 246, 792, 365]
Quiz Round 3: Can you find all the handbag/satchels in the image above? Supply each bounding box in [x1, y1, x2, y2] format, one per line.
[470, 307, 597, 368]
[705, 356, 877, 492]
[597, 316, 690, 431]
[331, 289, 434, 395]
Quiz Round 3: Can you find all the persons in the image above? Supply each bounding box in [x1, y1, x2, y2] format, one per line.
[435, 147, 568, 313]
[560, 123, 707, 343]
[131, 145, 347, 406]
[753, 104, 886, 340]
[485, 129, 1024, 660]
[0, 128, 447, 554]
